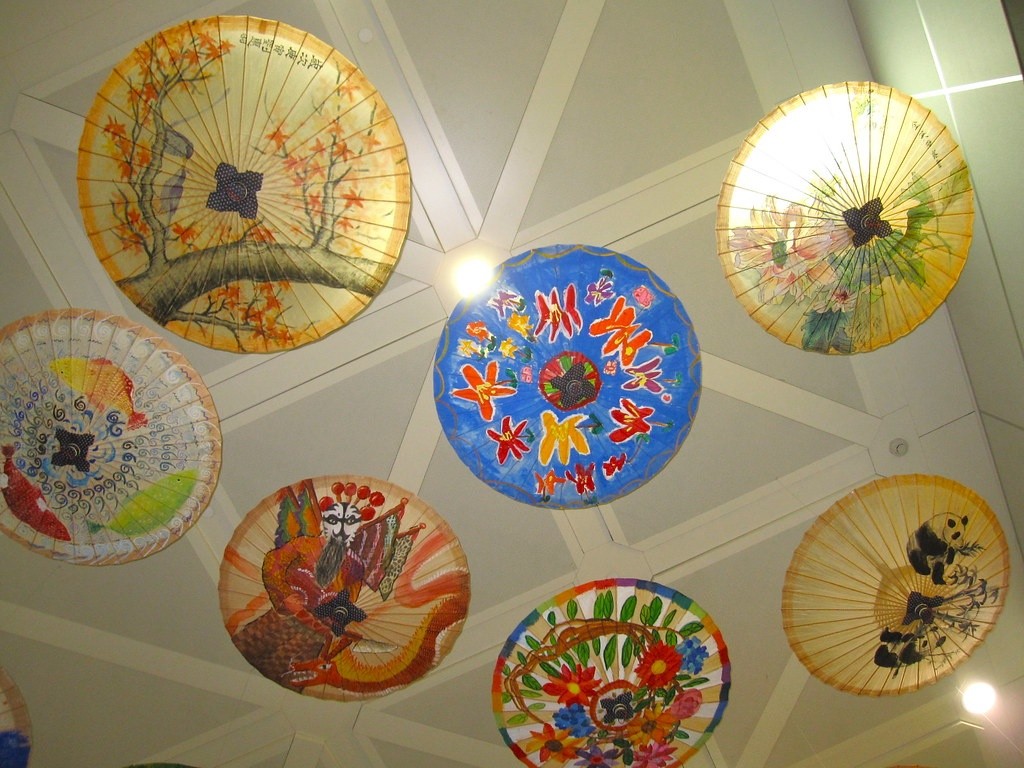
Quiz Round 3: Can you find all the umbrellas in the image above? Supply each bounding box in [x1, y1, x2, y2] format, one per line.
[434, 243, 707, 511]
[218, 475, 470, 703]
[75, 14, 413, 354]
[780, 474, 1011, 698]
[716, 82, 977, 358]
[492, 577, 731, 768]
[1, 306, 224, 568]
[0, 665, 34, 768]
